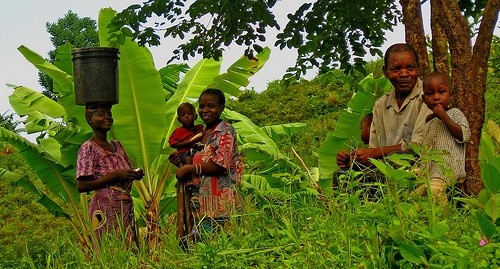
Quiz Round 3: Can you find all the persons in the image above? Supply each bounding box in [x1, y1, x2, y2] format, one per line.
[333, 43, 471, 206]
[76, 103, 145, 254]
[168, 89, 243, 240]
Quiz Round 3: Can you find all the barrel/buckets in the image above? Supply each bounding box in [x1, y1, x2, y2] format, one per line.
[72, 47, 120, 105]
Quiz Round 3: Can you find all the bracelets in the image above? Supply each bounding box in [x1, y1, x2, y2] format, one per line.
[199, 163, 201, 175]
[196, 164, 198, 174]
